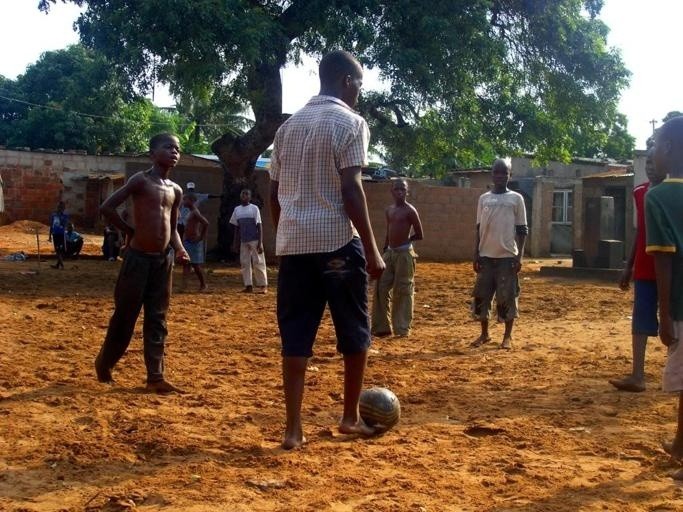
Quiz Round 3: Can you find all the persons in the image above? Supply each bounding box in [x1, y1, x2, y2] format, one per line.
[372, 179, 423, 337]
[47, 182, 226, 292]
[229, 188, 268, 295]
[94, 133, 191, 393]
[268, 50, 386, 449]
[643, 117, 682, 482]
[610, 146, 668, 392]
[469, 159, 529, 350]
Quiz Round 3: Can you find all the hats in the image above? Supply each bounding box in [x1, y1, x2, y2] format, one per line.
[186, 182, 195, 189]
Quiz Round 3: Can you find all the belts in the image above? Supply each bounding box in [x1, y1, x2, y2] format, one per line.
[129, 243, 171, 259]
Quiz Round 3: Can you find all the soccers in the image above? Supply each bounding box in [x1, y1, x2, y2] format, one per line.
[359, 388, 401, 433]
